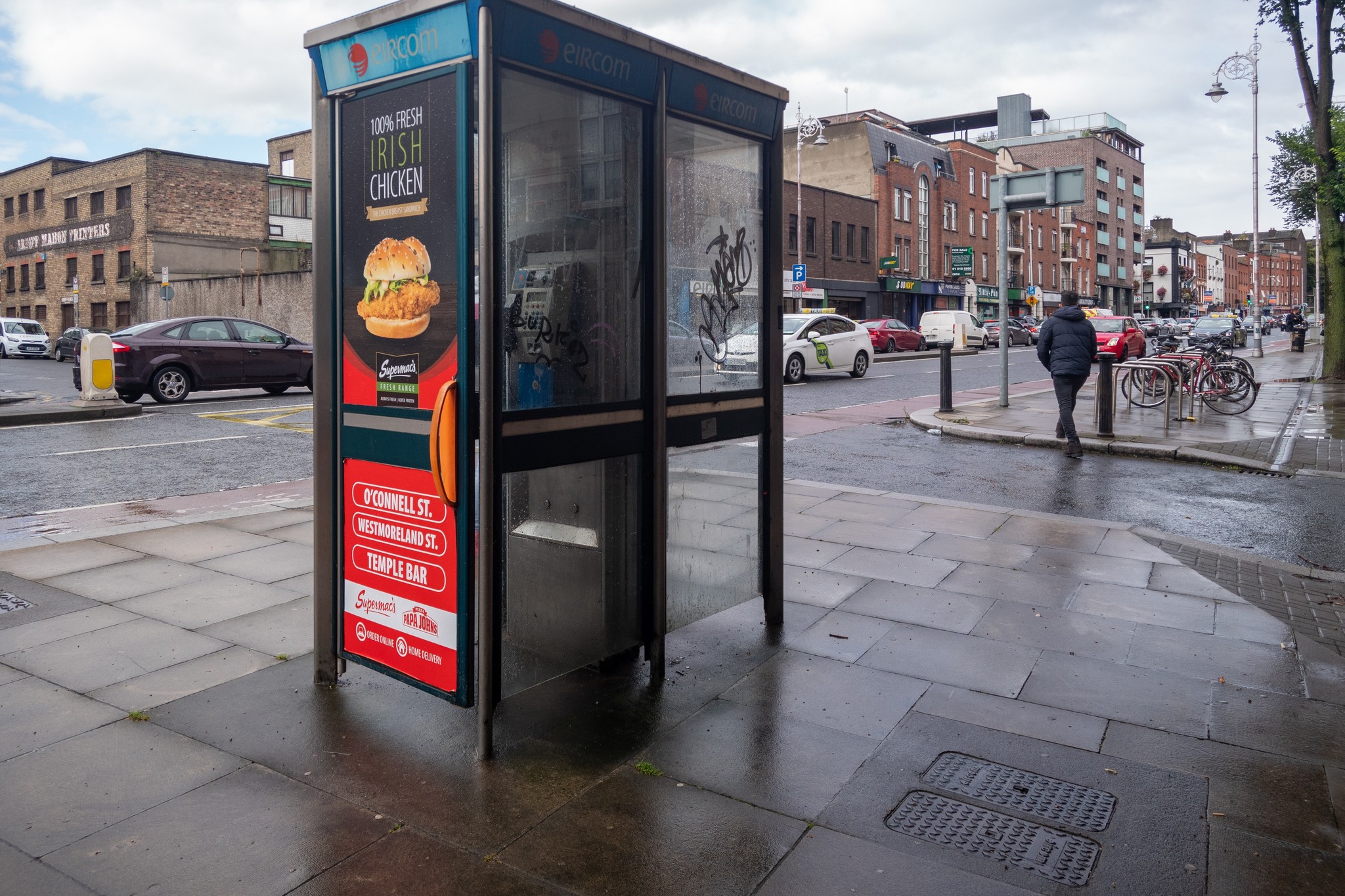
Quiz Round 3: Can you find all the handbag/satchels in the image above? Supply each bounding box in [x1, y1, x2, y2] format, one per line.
[1320, 328, 1324, 336]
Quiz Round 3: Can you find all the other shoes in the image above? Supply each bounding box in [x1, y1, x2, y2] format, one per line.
[1062, 440, 1084, 458]
[1056, 427, 1065, 438]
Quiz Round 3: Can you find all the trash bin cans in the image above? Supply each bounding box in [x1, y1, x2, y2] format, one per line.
[1156, 336, 1179, 378]
[1290, 326, 1306, 352]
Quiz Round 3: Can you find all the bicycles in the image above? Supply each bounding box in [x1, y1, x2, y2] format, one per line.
[1120, 333, 1262, 415]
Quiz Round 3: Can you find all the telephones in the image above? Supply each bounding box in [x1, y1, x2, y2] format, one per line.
[503, 261, 580, 366]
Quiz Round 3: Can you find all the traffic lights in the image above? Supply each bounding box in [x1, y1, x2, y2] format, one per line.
[1249, 295, 1253, 306]
[1146, 301, 1150, 309]
[1304, 306, 1305, 310]
[1301, 306, 1303, 311]
[1247, 294, 1251, 303]
[1030, 297, 1034, 305]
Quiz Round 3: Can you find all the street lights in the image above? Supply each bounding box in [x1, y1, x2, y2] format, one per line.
[1140, 218, 1158, 318]
[1286, 162, 1321, 328]
[795, 101, 830, 313]
[1204, 28, 1264, 358]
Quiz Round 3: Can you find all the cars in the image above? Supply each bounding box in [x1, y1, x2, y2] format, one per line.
[1030, 319, 1047, 345]
[1133, 311, 1325, 349]
[54, 327, 114, 362]
[713, 307, 874, 384]
[668, 320, 714, 365]
[857, 318, 926, 354]
[979, 319, 1033, 348]
[71, 316, 314, 405]
[1087, 315, 1146, 363]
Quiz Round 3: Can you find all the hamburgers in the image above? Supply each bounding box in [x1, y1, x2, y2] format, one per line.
[357, 236, 440, 339]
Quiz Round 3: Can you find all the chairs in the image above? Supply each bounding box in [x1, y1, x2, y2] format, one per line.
[194, 327, 249, 341]
[1101, 324, 1137, 333]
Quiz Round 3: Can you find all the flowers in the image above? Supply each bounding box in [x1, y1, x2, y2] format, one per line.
[1156, 285, 1168, 303]
[1156, 263, 1168, 277]
[1142, 267, 1153, 286]
[1178, 264, 1195, 283]
[1132, 279, 1142, 296]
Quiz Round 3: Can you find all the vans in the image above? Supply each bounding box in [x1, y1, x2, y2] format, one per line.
[918, 310, 989, 350]
[1081, 309, 1119, 328]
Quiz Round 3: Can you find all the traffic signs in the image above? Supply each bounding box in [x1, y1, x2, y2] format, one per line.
[1028, 286, 1035, 295]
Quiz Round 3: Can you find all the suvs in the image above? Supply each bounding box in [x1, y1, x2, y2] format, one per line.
[0, 317, 52, 360]
[1008, 315, 1039, 330]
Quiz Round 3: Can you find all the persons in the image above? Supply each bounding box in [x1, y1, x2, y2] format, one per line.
[1037, 291, 1097, 458]
[1185, 315, 1188, 318]
[1286, 307, 1304, 352]
[1150, 314, 1155, 318]
[1319, 317, 1325, 326]
